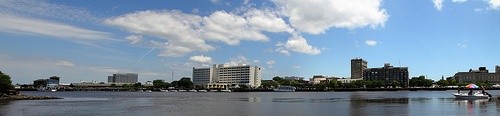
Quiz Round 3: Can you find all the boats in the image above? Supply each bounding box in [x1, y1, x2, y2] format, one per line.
[452, 91, 489, 100]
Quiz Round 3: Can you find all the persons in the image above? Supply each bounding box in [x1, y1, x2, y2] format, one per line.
[457, 87, 461, 94]
[468, 89, 473, 95]
[480, 85, 486, 95]
